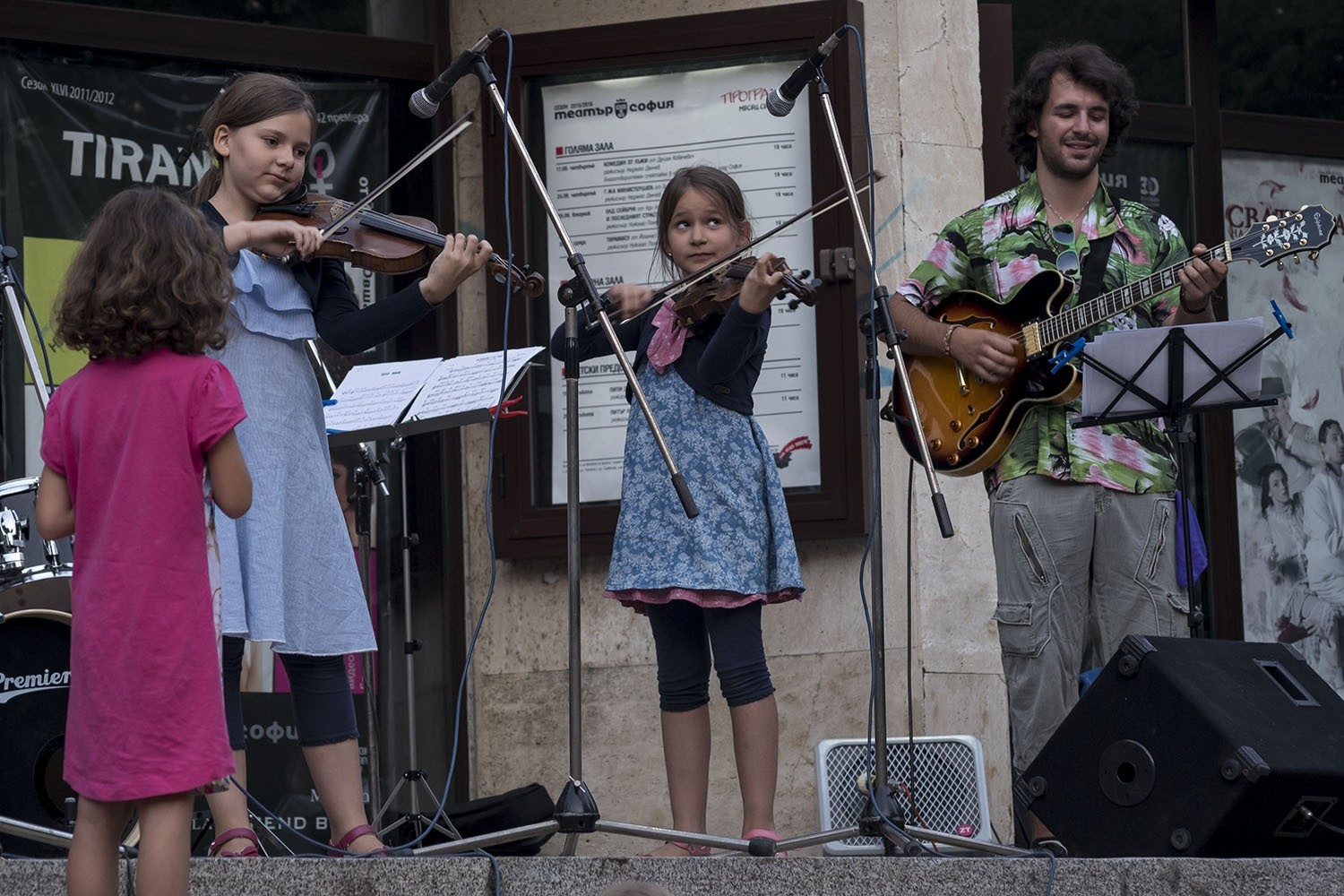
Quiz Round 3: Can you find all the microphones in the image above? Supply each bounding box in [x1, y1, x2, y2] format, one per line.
[763, 22, 852, 119]
[406, 25, 505, 120]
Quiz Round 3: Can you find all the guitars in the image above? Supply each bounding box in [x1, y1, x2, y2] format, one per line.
[891, 204, 1340, 479]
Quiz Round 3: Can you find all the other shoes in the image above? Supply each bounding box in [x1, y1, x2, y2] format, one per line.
[1030, 837, 1070, 857]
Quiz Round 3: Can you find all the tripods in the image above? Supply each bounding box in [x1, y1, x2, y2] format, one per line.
[413, 62, 770, 858]
[327, 361, 531, 852]
[772, 68, 1055, 864]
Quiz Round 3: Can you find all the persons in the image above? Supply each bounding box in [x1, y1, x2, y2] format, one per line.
[182, 74, 492, 858]
[1236, 377, 1344, 698]
[37, 187, 253, 895]
[551, 163, 794, 856]
[885, 43, 1230, 859]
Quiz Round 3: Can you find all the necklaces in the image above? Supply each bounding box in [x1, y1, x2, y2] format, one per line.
[1043, 196, 1094, 224]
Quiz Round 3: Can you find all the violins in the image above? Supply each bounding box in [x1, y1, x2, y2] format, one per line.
[671, 254, 825, 325]
[246, 190, 548, 298]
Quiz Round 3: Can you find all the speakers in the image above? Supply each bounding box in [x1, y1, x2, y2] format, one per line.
[1010, 631, 1344, 858]
[816, 732, 993, 857]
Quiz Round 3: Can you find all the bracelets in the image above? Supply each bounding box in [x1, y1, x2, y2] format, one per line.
[944, 324, 965, 356]
[1180, 286, 1210, 314]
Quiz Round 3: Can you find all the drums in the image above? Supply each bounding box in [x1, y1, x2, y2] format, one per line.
[0, 604, 142, 859]
[1, 474, 78, 615]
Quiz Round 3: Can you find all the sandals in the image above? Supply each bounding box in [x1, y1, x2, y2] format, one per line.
[209, 828, 261, 856]
[326, 824, 388, 857]
[743, 829, 788, 858]
[635, 841, 713, 856]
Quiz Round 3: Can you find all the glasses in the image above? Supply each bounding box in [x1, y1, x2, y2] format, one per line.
[1052, 221, 1080, 280]
